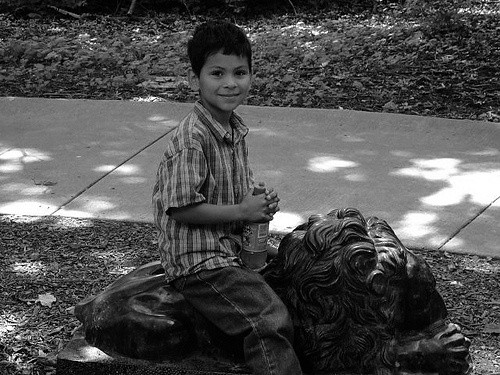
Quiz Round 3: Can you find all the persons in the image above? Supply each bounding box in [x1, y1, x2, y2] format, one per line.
[152, 19, 303, 374]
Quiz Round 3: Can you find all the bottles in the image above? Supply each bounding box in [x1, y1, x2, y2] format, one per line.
[242, 182, 269, 269]
